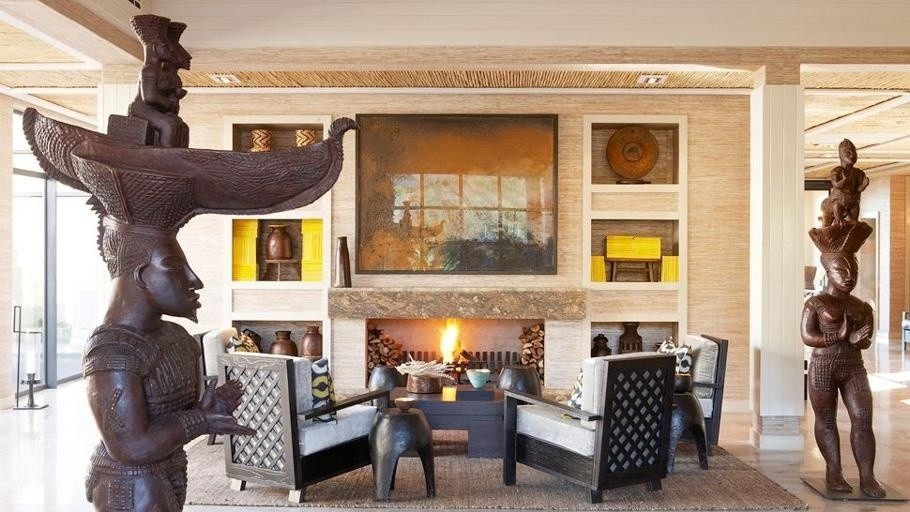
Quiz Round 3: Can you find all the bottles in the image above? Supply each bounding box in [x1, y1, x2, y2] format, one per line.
[266, 225, 353, 356]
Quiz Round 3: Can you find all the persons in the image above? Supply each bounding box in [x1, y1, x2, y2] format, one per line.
[159, 20, 191, 148]
[127, 13, 182, 148]
[820, 137, 870, 224]
[84, 219, 256, 512]
[798, 252, 887, 499]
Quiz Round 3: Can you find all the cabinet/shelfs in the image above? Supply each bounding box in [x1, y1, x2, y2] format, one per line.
[227, 118, 329, 362]
[578, 110, 691, 359]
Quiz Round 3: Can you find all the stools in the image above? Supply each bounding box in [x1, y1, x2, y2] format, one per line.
[495, 364, 542, 397]
[367, 408, 435, 502]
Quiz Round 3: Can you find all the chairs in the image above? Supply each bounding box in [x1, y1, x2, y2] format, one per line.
[502, 333, 730, 504]
[193, 326, 392, 506]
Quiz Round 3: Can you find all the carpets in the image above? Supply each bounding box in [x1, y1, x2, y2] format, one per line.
[167, 439, 810, 512]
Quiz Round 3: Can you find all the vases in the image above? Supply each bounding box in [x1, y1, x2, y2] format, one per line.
[263, 222, 293, 260]
[336, 234, 351, 288]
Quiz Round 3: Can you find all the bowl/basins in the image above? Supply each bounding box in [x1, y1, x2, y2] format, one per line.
[466, 369, 491, 388]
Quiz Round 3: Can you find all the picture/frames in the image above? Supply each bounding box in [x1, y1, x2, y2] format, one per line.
[352, 115, 561, 275]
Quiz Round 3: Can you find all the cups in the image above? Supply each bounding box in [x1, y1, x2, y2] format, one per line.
[394, 397, 416, 411]
